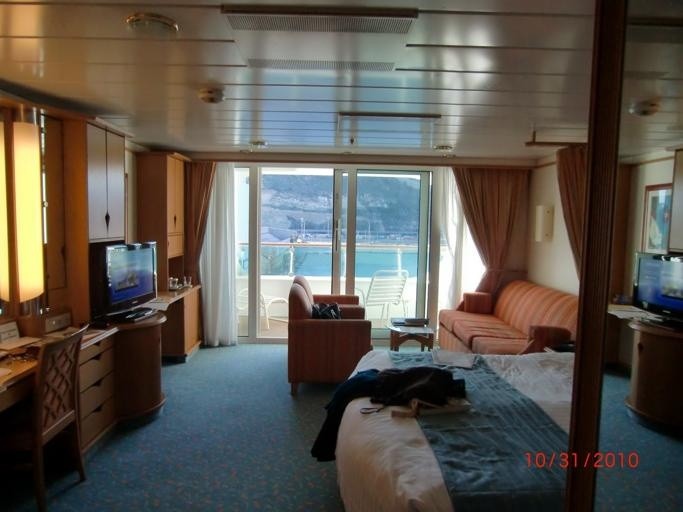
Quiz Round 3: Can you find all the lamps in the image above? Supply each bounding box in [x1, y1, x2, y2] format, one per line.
[0, 102, 45, 303]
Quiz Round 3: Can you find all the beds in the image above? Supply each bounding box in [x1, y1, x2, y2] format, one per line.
[335, 350, 683, 511]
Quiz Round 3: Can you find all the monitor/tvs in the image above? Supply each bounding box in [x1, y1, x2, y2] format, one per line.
[105, 241, 158, 324]
[632, 252, 683, 330]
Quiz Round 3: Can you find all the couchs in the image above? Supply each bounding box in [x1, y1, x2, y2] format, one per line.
[438, 279, 580, 355]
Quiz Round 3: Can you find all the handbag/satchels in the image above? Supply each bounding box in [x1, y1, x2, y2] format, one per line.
[312, 303, 341, 319]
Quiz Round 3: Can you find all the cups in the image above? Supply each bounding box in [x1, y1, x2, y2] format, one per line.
[170, 278, 179, 288]
[183, 276, 192, 286]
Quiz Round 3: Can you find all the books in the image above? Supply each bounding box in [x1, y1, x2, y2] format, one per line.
[405, 317, 424, 327]
[418, 400, 471, 416]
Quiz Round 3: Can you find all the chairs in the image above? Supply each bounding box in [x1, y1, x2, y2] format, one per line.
[355, 269, 407, 328]
[293, 275, 359, 305]
[0, 324, 86, 511]
[236, 288, 288, 329]
[288, 283, 373, 396]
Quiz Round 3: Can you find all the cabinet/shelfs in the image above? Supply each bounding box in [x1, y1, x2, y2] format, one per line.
[84, 118, 125, 243]
[166, 156, 185, 259]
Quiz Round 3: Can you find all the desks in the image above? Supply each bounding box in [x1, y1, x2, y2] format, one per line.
[0, 327, 118, 464]
[386, 321, 434, 352]
[91, 285, 201, 427]
